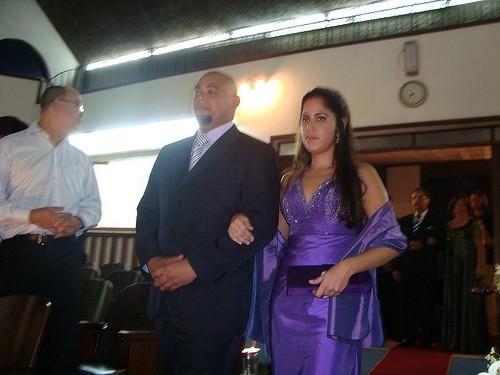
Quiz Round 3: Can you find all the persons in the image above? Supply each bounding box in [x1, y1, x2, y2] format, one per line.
[227, 88, 408, 374]
[469, 190, 496, 265]
[391, 188, 447, 351]
[134, 72, 282, 375]
[427, 194, 492, 354]
[0, 85, 102, 375]
[0, 116, 29, 142]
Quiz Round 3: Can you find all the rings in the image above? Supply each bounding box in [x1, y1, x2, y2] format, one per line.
[320, 270, 328, 277]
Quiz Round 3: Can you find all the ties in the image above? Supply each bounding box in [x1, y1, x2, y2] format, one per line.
[185, 132, 209, 174]
[411, 214, 422, 238]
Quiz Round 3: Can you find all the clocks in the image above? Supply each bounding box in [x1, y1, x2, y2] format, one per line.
[400, 80, 428, 107]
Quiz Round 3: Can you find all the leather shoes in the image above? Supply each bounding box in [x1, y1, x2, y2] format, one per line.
[422, 340, 435, 349]
[400, 338, 418, 348]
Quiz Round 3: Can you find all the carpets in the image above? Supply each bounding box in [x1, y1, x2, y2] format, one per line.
[354, 348, 500, 375]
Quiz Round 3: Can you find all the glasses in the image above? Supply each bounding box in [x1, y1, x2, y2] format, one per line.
[453, 202, 469, 209]
[410, 196, 428, 202]
[51, 98, 84, 113]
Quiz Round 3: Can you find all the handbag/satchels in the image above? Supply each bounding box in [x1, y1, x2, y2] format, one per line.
[285, 263, 373, 298]
[468, 272, 492, 294]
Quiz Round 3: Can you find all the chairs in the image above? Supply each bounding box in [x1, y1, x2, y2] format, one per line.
[0, 263, 173, 375]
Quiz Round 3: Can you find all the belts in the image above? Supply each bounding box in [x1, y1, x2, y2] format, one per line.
[16, 232, 79, 247]
